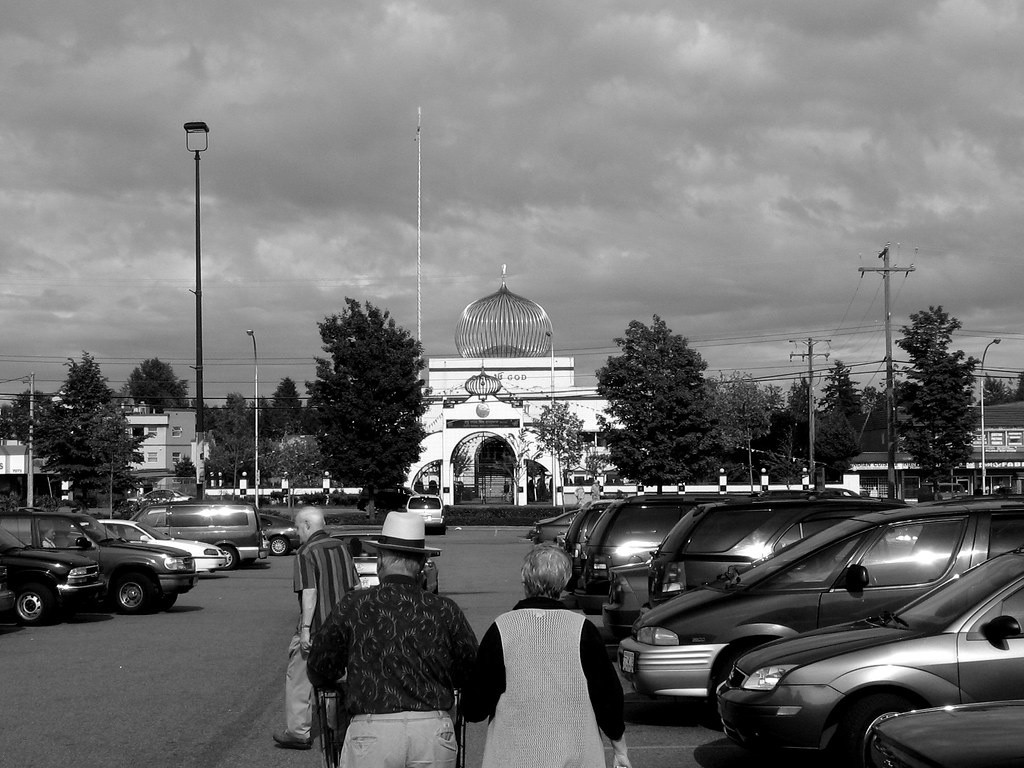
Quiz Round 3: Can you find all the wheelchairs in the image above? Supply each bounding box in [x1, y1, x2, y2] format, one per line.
[311, 679, 467, 768]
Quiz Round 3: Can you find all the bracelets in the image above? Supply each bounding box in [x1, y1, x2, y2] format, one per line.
[301, 624, 309, 628]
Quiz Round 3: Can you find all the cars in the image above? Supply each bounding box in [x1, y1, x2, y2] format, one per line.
[259, 511, 302, 556]
[356, 485, 419, 512]
[125, 489, 194, 509]
[717, 543, 1024, 768]
[71, 518, 227, 573]
[862, 698, 1024, 768]
[0, 526, 107, 627]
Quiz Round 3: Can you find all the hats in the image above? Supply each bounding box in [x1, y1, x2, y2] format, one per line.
[359, 510, 444, 552]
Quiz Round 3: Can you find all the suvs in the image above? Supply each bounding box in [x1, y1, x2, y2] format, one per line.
[117, 501, 271, 572]
[407, 493, 446, 534]
[0, 507, 198, 615]
[616, 492, 1024, 731]
[525, 487, 916, 644]
[934, 483, 969, 502]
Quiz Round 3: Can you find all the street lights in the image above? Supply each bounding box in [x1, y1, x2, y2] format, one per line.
[545, 331, 557, 508]
[183, 121, 210, 506]
[981, 336, 1002, 497]
[244, 329, 260, 509]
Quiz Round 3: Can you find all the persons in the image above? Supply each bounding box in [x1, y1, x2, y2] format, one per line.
[349, 538, 365, 552]
[306, 511, 480, 767]
[273, 507, 360, 746]
[42, 525, 57, 548]
[462, 542, 633, 768]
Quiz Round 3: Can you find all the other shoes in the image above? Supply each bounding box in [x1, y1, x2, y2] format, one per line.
[273, 729, 314, 750]
